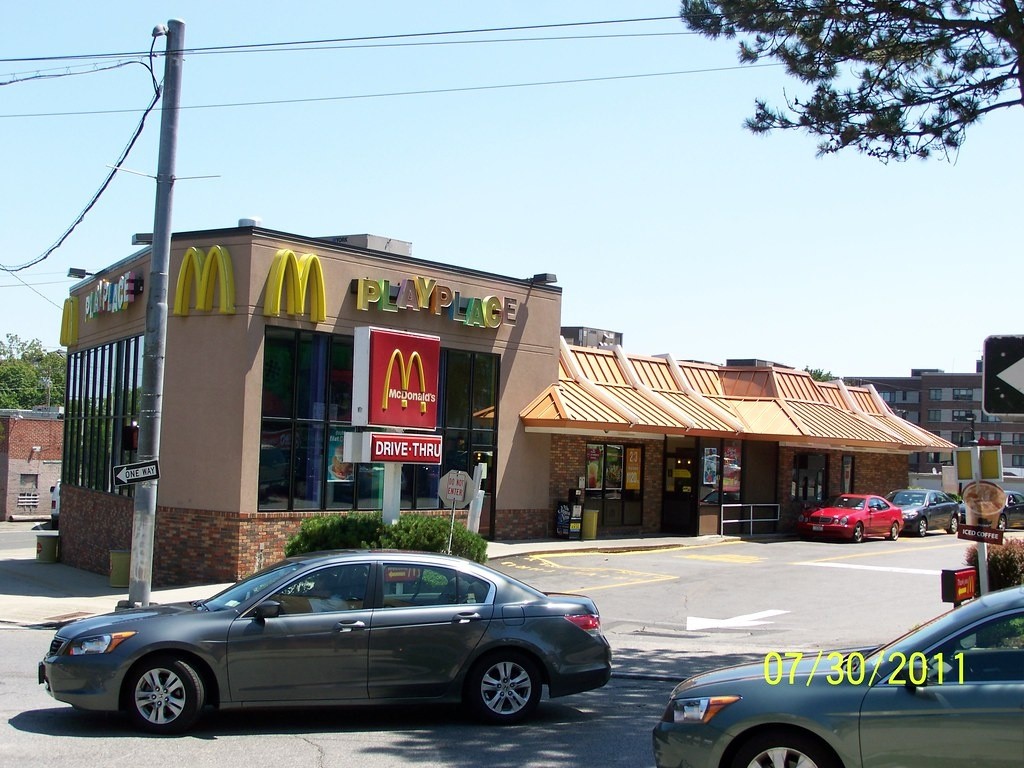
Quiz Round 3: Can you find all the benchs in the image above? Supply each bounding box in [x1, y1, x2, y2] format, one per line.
[437, 577, 487, 604]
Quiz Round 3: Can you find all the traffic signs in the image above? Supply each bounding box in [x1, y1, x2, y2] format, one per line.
[113, 460, 160, 487]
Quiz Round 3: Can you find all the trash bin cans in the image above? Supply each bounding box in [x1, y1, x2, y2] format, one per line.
[109, 549, 130, 587]
[582, 509, 599, 539]
[556, 489, 584, 540]
[36, 534, 59, 563]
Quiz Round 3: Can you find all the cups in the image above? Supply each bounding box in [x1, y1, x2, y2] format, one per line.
[961, 482, 1007, 529]
[588, 464, 597, 488]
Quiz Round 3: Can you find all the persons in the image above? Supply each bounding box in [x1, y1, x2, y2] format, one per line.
[269, 572, 356, 614]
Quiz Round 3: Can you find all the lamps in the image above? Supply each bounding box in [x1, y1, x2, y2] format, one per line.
[67, 268, 93, 280]
[601, 334, 607, 343]
[532, 272, 557, 284]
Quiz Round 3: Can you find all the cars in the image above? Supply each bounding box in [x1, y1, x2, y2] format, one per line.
[874, 488, 961, 537]
[794, 490, 905, 544]
[958, 490, 1024, 531]
[50, 477, 61, 530]
[651, 583, 1024, 768]
[258, 439, 494, 504]
[699, 485, 740, 504]
[37, 547, 613, 738]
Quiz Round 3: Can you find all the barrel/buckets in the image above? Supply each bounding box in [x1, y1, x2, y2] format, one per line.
[581, 508, 599, 540]
